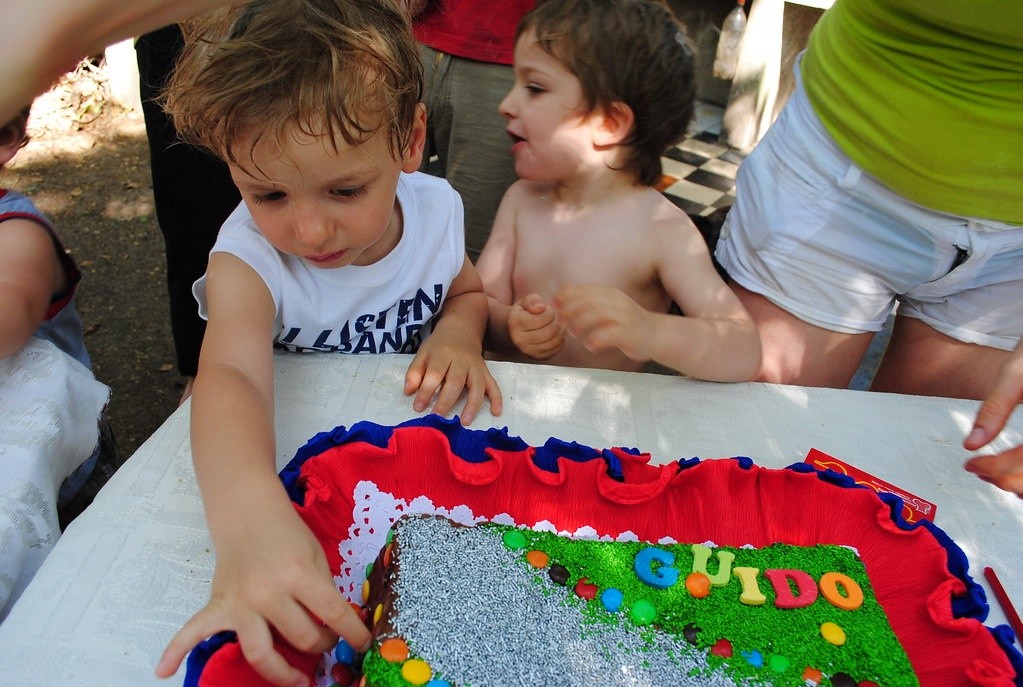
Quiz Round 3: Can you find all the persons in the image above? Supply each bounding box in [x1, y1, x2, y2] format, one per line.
[476, 0, 763, 382]
[133, 23, 242, 408]
[156, 0, 503, 687]
[0, 0, 198, 127]
[713, 0, 1023, 499]
[0, 99, 101, 517]
[395, 0, 540, 267]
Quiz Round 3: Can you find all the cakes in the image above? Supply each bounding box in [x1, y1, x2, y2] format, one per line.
[331, 511, 921, 687]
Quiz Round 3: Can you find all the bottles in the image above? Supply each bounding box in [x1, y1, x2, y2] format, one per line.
[712, 0, 747, 78]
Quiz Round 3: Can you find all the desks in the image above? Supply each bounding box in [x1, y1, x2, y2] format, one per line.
[0, 344, 1023, 687]
